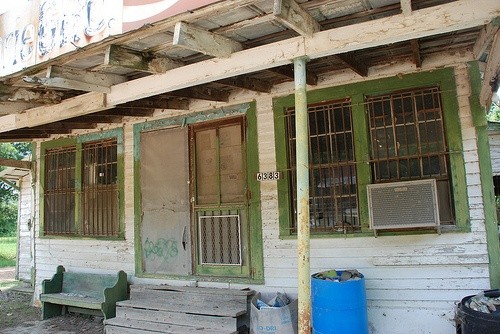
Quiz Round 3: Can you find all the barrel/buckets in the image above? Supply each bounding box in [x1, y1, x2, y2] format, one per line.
[460, 288, 499, 334]
[312, 269, 368, 334]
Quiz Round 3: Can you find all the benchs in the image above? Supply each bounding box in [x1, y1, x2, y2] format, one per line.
[39, 265, 129, 320]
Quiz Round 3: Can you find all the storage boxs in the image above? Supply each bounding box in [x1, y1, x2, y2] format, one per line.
[249, 291, 298, 334]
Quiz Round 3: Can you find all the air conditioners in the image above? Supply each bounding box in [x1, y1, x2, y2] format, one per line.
[367, 178, 441, 238]
